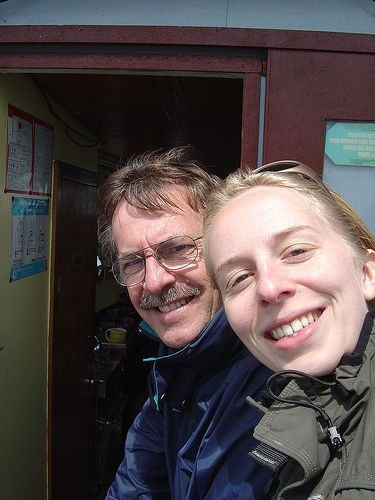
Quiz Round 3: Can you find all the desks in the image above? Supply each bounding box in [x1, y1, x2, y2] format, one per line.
[87, 342, 127, 461]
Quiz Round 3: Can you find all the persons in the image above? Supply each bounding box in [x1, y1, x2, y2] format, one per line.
[203, 161, 375, 500]
[97, 149, 275, 500]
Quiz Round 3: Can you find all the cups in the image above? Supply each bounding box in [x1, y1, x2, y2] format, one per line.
[106, 328, 127, 344]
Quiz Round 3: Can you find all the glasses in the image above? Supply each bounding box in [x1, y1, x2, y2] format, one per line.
[110, 236, 207, 287]
[251, 160, 336, 203]
